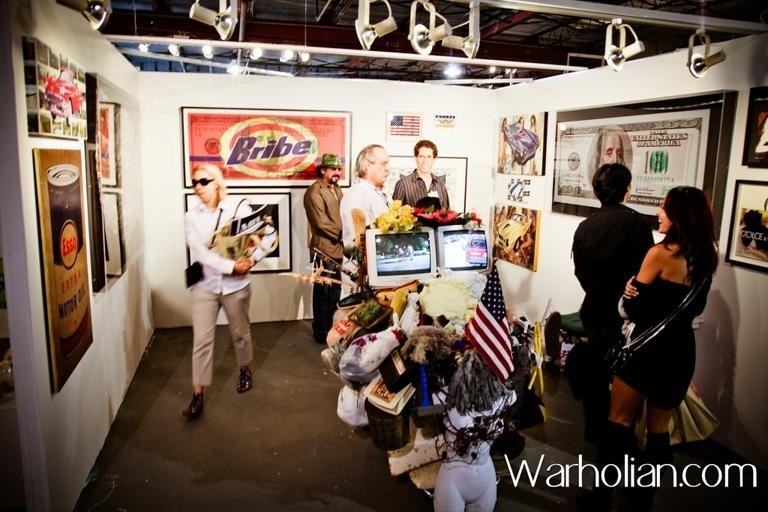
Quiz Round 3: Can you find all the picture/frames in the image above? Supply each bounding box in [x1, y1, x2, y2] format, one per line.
[551, 89, 738, 240]
[741, 85, 768, 168]
[30, 148, 94, 399]
[385, 157, 468, 216]
[180, 106, 353, 190]
[184, 193, 293, 274]
[724, 179, 768, 274]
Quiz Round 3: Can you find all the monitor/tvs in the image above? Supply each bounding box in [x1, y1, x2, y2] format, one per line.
[435, 224, 490, 273]
[365, 227, 438, 287]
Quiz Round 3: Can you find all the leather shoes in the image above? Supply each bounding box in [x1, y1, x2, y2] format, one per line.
[235, 367, 252, 393]
[182, 392, 205, 418]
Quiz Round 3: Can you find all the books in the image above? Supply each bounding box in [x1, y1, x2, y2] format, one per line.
[229, 203, 270, 236]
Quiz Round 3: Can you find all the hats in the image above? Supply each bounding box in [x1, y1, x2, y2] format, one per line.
[320, 153, 342, 168]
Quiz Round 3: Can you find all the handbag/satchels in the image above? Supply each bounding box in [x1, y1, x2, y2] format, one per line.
[679, 382, 720, 446]
[184, 208, 223, 288]
[602, 280, 708, 372]
[667, 410, 682, 446]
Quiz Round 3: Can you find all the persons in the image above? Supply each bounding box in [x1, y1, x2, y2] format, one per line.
[180, 163, 276, 422]
[518, 117, 526, 174]
[570, 163, 654, 463]
[393, 140, 450, 212]
[495, 206, 506, 229]
[594, 130, 626, 173]
[499, 118, 507, 164]
[430, 353, 507, 512]
[336, 144, 393, 302]
[519, 210, 536, 268]
[605, 185, 719, 473]
[303, 153, 344, 344]
[528, 115, 538, 175]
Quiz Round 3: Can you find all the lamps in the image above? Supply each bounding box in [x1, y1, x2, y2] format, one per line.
[604, 20, 644, 70]
[686, 29, 729, 76]
[354, 1, 396, 48]
[190, 1, 239, 41]
[444, 4, 482, 61]
[406, 1, 453, 55]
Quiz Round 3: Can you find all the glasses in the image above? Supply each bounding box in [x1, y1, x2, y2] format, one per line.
[330, 167, 342, 171]
[191, 177, 214, 187]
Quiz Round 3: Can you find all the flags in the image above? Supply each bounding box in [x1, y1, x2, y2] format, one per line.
[390, 115, 420, 136]
[464, 264, 515, 383]
